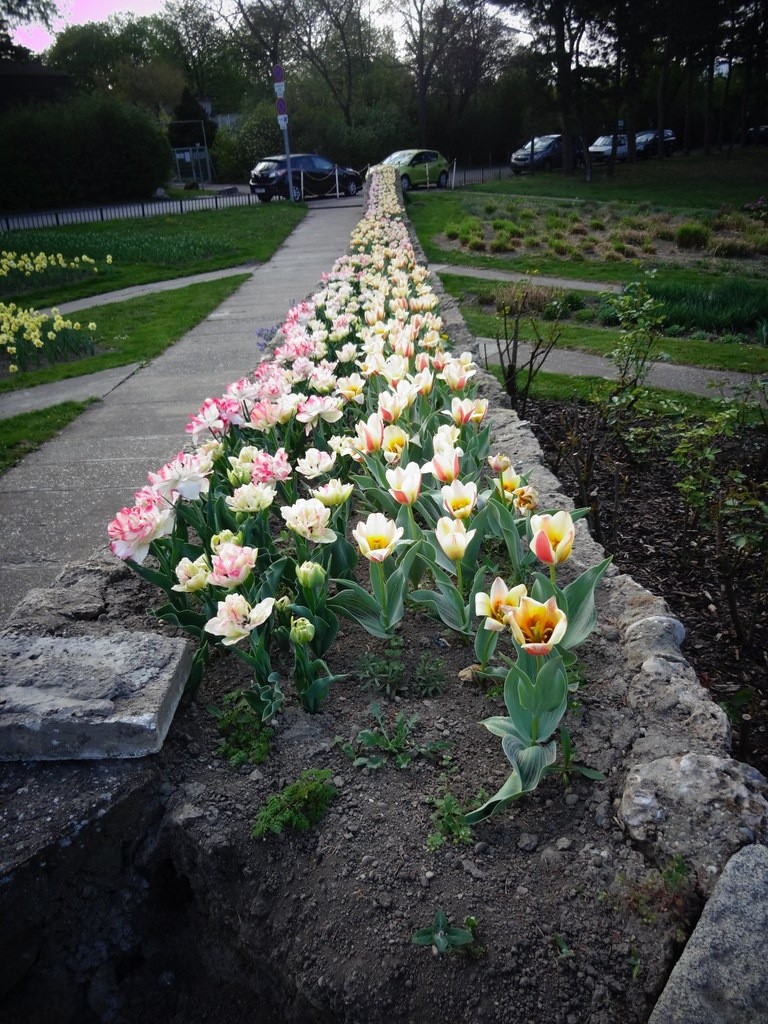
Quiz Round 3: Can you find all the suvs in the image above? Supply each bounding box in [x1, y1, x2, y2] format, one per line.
[249, 153, 363, 203]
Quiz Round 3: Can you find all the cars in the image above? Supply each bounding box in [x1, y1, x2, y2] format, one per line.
[364, 148, 450, 192]
[588, 133, 629, 164]
[743, 126, 768, 146]
[510, 133, 573, 176]
[632, 130, 677, 159]
